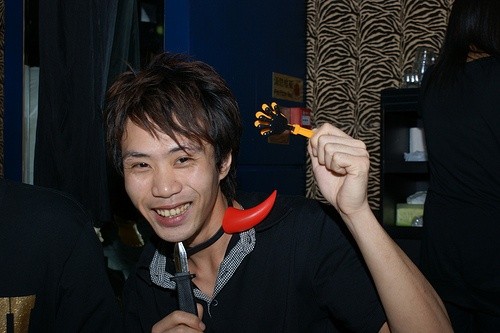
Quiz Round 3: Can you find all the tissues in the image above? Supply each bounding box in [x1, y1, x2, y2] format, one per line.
[396, 191, 427, 227]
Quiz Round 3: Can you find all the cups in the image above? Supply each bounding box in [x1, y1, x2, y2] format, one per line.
[404, 47, 436, 88]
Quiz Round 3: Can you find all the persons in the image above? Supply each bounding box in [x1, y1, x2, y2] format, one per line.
[418, 0, 500, 333]
[103, 52, 453, 333]
[0, 179, 119, 333]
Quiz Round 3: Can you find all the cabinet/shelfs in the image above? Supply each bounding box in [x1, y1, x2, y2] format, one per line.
[377, 88, 429, 240]
